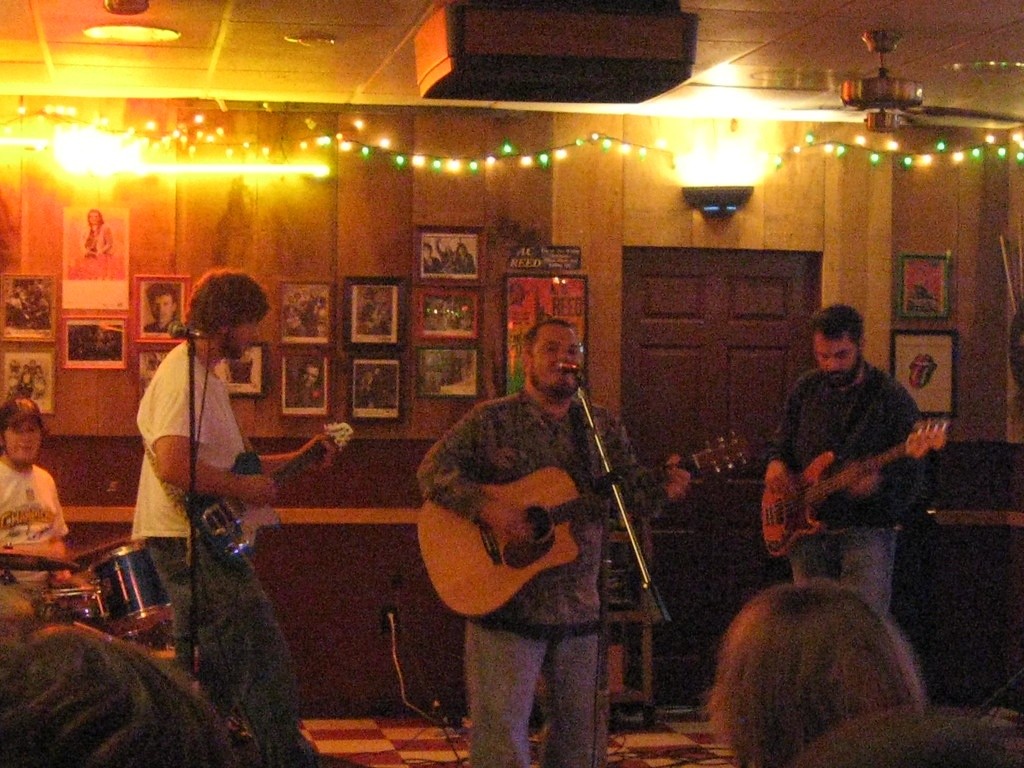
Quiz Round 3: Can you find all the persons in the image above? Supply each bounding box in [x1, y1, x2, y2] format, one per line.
[83, 209, 113, 257]
[422, 239, 475, 274]
[132, 266, 342, 768]
[0, 584, 237, 768]
[714, 584, 1024, 767]
[759, 303, 929, 620]
[287, 359, 324, 407]
[144, 283, 183, 332]
[416, 318, 692, 768]
[0, 396, 93, 590]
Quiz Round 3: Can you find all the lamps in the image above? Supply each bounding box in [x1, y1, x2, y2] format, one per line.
[683, 186, 755, 219]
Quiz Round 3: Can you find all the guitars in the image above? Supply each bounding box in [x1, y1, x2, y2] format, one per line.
[760, 419, 951, 557]
[186, 419, 354, 564]
[413, 426, 755, 615]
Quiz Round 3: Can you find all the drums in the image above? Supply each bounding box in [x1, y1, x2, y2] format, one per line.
[41, 586, 109, 623]
[89, 542, 176, 636]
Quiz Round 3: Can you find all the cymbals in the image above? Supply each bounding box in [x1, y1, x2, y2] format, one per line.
[1, 553, 81, 570]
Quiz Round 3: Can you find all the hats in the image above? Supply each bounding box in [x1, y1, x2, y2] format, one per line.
[1, 398, 43, 428]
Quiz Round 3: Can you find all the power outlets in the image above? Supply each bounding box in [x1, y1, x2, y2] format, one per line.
[384, 606, 398, 633]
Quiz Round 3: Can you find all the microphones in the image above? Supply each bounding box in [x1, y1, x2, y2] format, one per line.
[166, 320, 203, 340]
[557, 357, 580, 374]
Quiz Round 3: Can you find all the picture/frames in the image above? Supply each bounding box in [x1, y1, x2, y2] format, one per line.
[60, 314, 129, 369]
[278, 279, 335, 348]
[349, 356, 408, 426]
[0, 348, 57, 415]
[279, 350, 332, 417]
[898, 253, 953, 319]
[342, 275, 405, 353]
[890, 328, 958, 420]
[501, 273, 590, 395]
[0, 273, 57, 344]
[134, 274, 189, 345]
[135, 349, 169, 395]
[419, 290, 483, 340]
[416, 345, 484, 400]
[411, 224, 484, 288]
